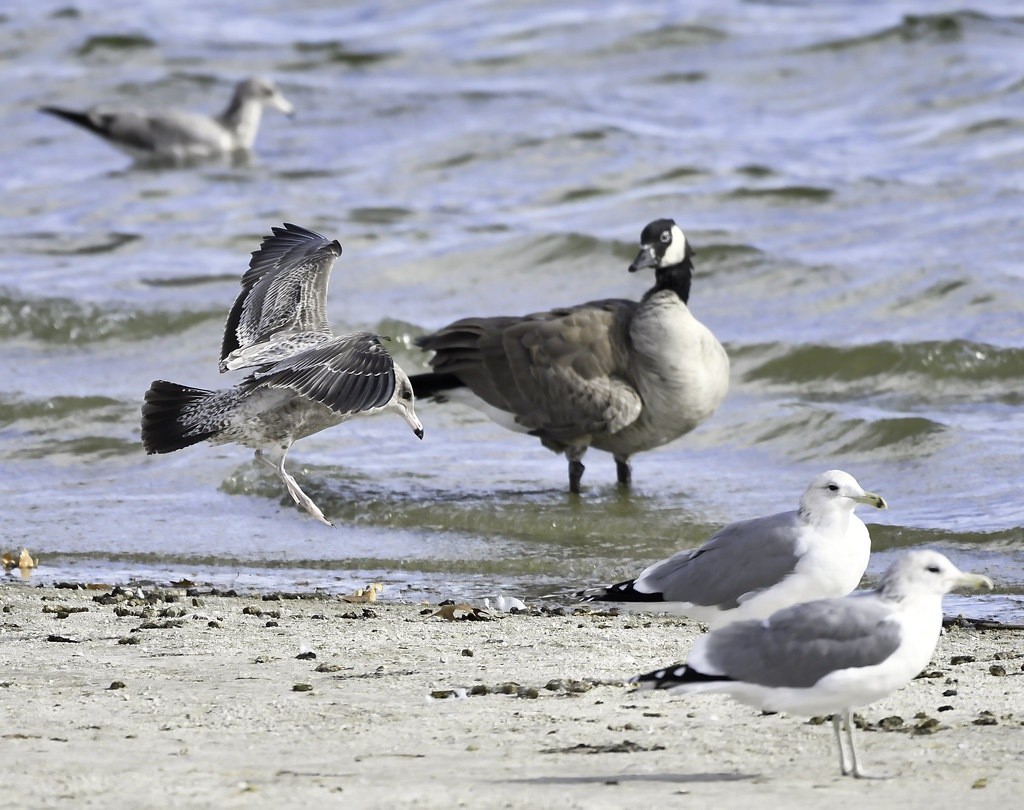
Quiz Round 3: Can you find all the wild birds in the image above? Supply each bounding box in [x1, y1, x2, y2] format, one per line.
[582, 467, 887, 629]
[629, 547, 995, 782]
[139, 225, 423, 531]
[50, 78, 297, 175]
[422, 216, 732, 494]
